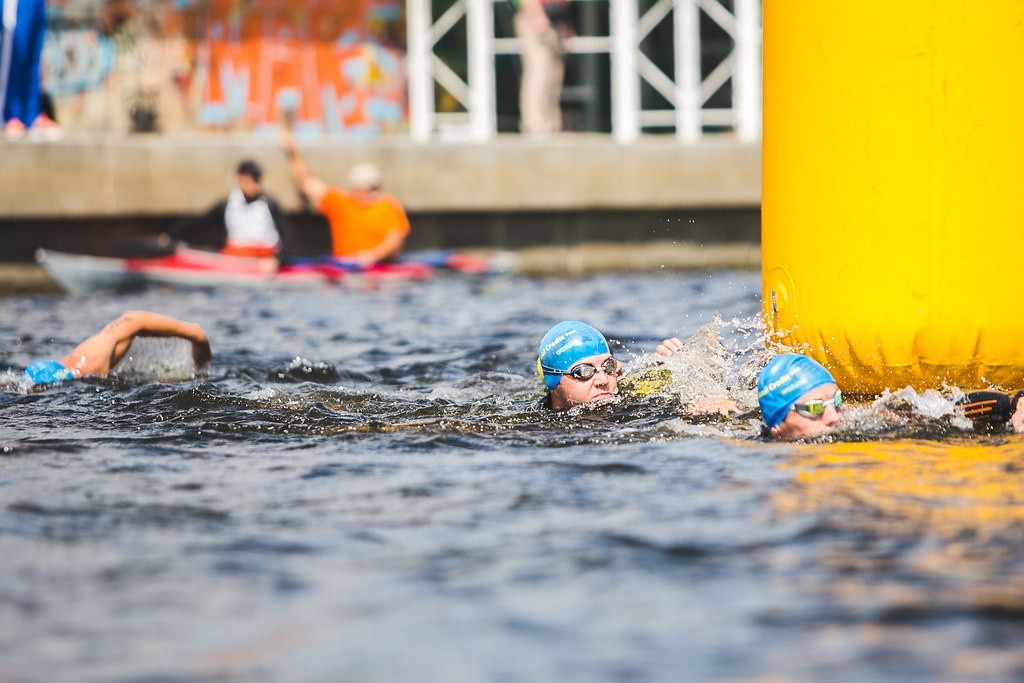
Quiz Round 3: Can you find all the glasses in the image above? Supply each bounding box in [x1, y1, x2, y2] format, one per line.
[791, 390, 842, 419]
[542, 356, 617, 382]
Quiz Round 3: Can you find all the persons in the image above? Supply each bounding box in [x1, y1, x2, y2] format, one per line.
[534, 321, 742, 418]
[160, 163, 295, 275]
[0, 0, 62, 142]
[512, 0, 573, 134]
[19, 310, 211, 396]
[749, 353, 1024, 444]
[279, 105, 409, 269]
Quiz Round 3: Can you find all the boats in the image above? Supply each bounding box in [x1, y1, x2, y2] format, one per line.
[33, 245, 531, 305]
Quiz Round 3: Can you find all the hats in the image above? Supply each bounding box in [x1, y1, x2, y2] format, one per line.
[758, 353, 836, 429]
[540, 321, 611, 390]
[21, 360, 75, 390]
